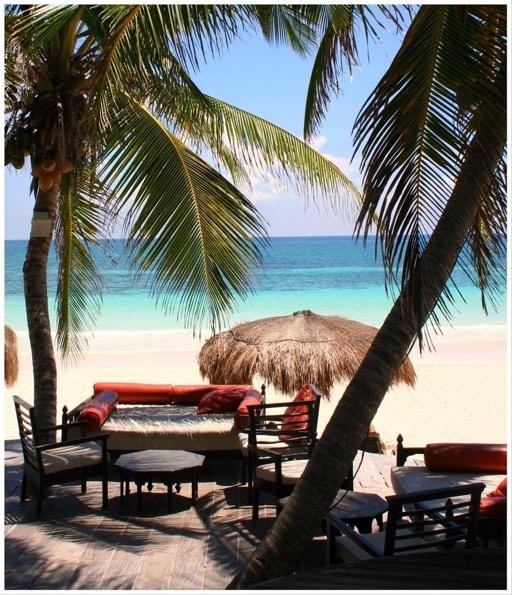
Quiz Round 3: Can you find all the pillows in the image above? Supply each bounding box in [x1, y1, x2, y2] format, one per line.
[424, 444, 507, 474]
[197, 387, 246, 414]
[486, 477, 507, 497]
[278, 381, 314, 445]
[78, 390, 119, 432]
[235, 389, 261, 431]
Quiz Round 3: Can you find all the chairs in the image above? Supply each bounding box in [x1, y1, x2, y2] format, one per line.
[326, 483, 484, 565]
[13, 395, 111, 517]
[238, 383, 321, 485]
[247, 399, 353, 520]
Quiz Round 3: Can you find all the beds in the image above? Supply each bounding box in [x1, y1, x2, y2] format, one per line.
[392, 434, 507, 547]
[62, 383, 265, 484]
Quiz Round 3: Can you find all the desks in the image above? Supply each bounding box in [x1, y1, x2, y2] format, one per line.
[276, 487, 390, 538]
[113, 449, 206, 507]
